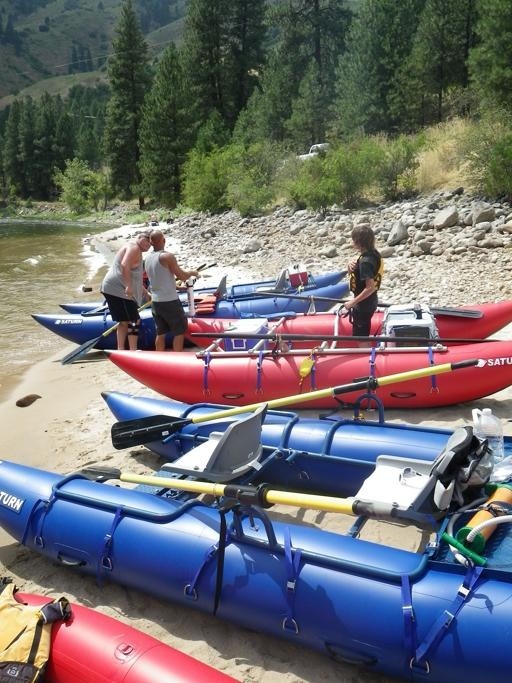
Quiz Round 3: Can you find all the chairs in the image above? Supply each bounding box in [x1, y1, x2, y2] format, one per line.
[160, 404, 268, 482]
[256, 269, 286, 293]
[198, 276, 227, 295]
[354, 425, 472, 527]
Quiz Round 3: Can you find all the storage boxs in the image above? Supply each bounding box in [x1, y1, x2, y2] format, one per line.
[223, 319, 268, 351]
[288, 264, 308, 287]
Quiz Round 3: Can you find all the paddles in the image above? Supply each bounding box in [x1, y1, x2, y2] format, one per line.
[61, 264, 215, 364]
[110, 360, 479, 450]
[83, 465, 430, 529]
[250, 291, 484, 319]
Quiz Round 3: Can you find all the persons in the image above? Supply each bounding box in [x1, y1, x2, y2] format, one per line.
[98, 231, 154, 349]
[343, 223, 385, 336]
[142, 229, 202, 349]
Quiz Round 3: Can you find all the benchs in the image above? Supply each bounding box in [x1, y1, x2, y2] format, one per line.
[386, 302, 435, 346]
